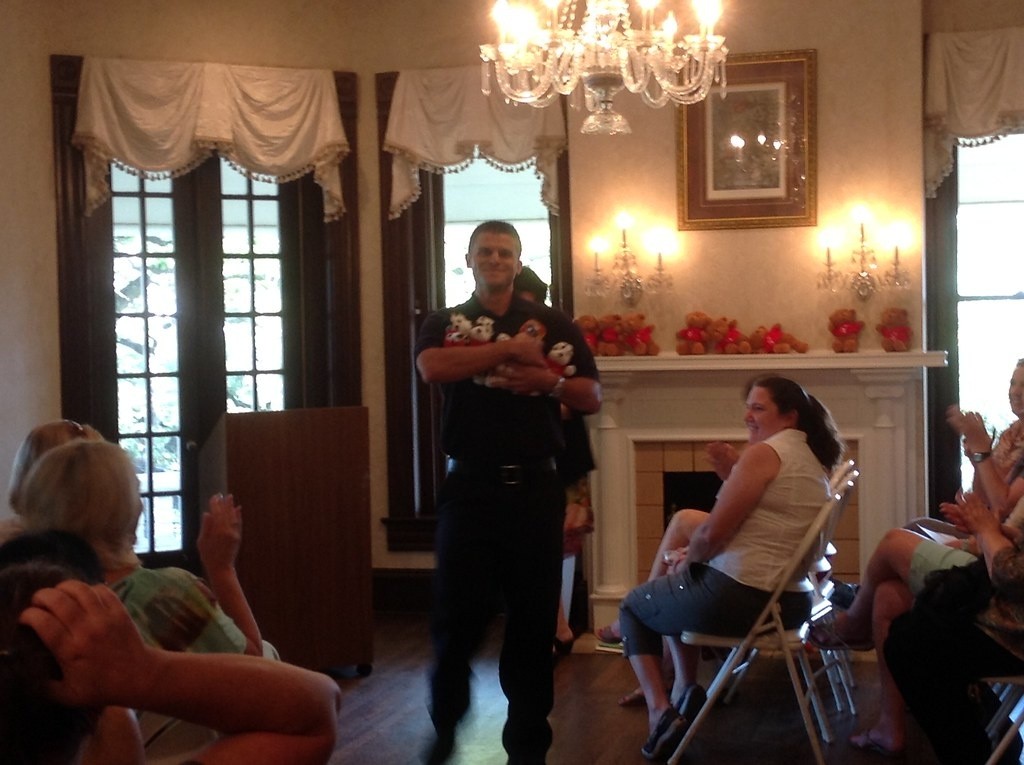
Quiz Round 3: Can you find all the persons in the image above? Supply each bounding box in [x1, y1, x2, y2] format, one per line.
[414, 222, 602, 765]
[0, 419, 345, 765]
[512, 265, 596, 660]
[595, 359, 1024, 765]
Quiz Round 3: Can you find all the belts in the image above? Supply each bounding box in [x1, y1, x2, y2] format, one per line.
[444, 456, 556, 486]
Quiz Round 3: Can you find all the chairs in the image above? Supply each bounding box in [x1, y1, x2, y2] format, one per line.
[981, 677, 1024, 765]
[667, 457, 861, 765]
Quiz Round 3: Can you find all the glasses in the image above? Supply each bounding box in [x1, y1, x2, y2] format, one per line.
[64, 419, 88, 439]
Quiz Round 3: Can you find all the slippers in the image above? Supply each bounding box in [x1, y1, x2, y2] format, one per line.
[808, 614, 876, 652]
[851, 729, 902, 758]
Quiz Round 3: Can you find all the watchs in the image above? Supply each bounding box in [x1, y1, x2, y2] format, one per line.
[969, 452, 993, 464]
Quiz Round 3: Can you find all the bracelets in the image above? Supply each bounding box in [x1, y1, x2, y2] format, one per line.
[962, 538, 968, 553]
[546, 377, 566, 397]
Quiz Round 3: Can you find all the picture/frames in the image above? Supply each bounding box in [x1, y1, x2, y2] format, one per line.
[674, 47, 818, 231]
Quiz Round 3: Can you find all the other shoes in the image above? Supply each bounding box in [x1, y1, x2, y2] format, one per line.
[617, 691, 647, 706]
[595, 625, 625, 644]
[676, 681, 707, 721]
[642, 706, 687, 761]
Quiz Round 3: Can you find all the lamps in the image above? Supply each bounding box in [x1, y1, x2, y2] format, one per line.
[815, 223, 914, 303]
[584, 227, 675, 307]
[479, 0, 730, 137]
